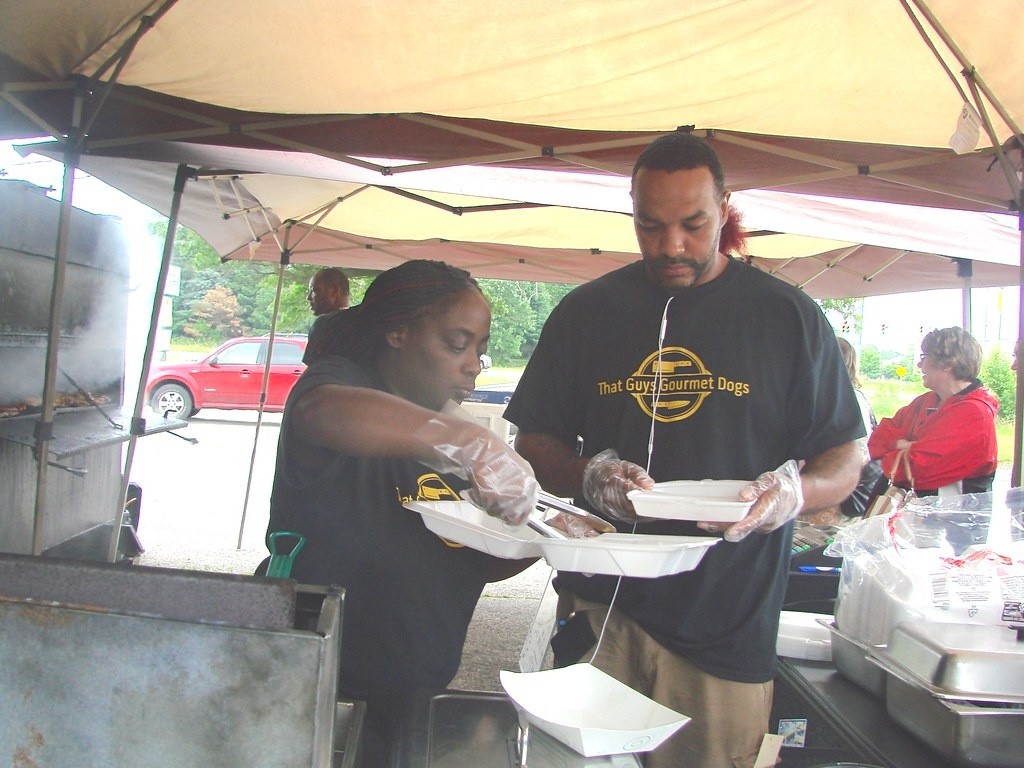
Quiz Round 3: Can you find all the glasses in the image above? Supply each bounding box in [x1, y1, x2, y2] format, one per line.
[920, 353, 933, 360]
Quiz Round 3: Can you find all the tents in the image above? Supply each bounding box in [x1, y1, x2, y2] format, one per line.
[0, 0, 1024, 576]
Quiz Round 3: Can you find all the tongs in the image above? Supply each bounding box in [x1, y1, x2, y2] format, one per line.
[523, 493, 616, 539]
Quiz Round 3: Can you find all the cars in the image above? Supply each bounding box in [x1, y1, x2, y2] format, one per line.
[480, 354, 492, 369]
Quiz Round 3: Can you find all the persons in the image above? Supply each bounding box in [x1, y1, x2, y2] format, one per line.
[1011, 337, 1020, 371]
[251, 259, 599, 768]
[868, 327, 1001, 555]
[502, 133, 868, 768]
[306, 268, 350, 317]
[837, 337, 878, 466]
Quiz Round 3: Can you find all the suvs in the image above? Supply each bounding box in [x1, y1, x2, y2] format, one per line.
[143, 332, 308, 422]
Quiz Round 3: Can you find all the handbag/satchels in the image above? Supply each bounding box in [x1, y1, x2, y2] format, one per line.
[863, 449, 919, 519]
[838, 458, 886, 518]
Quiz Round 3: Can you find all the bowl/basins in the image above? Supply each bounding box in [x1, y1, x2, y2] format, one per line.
[626, 479, 757, 522]
[401, 499, 722, 578]
